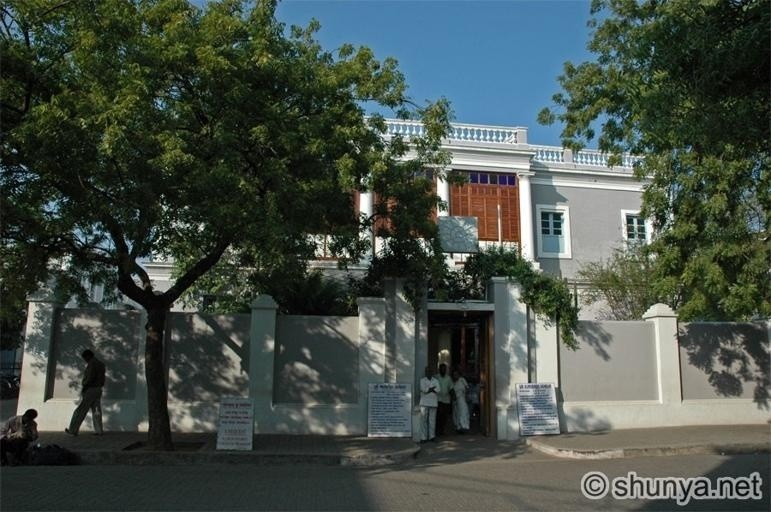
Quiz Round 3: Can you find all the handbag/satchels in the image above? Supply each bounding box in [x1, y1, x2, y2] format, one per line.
[30, 445, 66, 465]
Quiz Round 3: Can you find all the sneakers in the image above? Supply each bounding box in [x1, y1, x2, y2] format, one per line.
[65, 428, 76, 437]
[420, 428, 469, 444]
[1, 456, 21, 466]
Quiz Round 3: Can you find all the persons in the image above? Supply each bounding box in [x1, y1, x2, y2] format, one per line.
[420, 365, 441, 443]
[451, 368, 472, 434]
[65, 349, 106, 436]
[435, 363, 457, 436]
[468, 377, 482, 416]
[1, 408, 39, 466]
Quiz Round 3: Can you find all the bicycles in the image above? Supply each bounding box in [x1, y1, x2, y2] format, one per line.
[1, 371, 20, 400]
